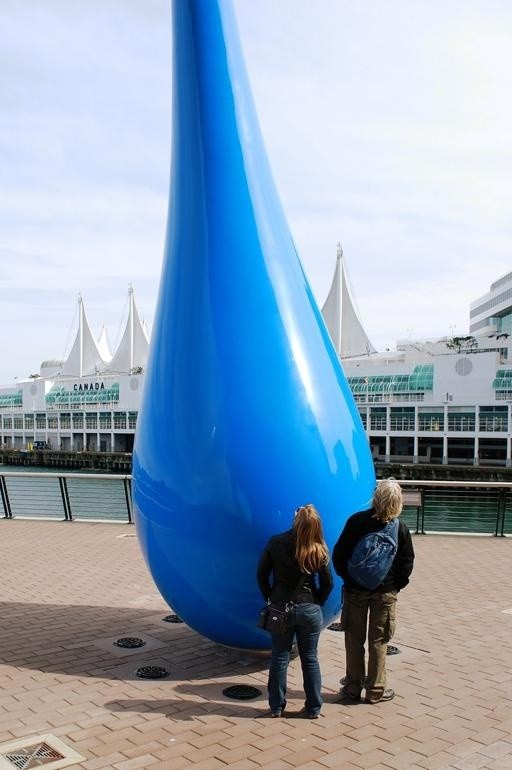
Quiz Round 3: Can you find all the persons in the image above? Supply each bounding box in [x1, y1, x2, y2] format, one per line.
[256, 506, 334, 718]
[332, 477, 415, 703]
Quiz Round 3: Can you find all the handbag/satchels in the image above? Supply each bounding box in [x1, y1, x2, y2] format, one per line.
[256, 604, 289, 635]
[348, 518, 399, 590]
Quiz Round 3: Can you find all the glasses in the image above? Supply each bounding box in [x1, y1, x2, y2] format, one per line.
[374, 487, 377, 491]
[296, 507, 304, 512]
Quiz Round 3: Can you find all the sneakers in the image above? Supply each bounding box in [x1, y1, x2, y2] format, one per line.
[309, 712, 321, 719]
[343, 685, 361, 701]
[365, 688, 395, 704]
[271, 710, 281, 718]
